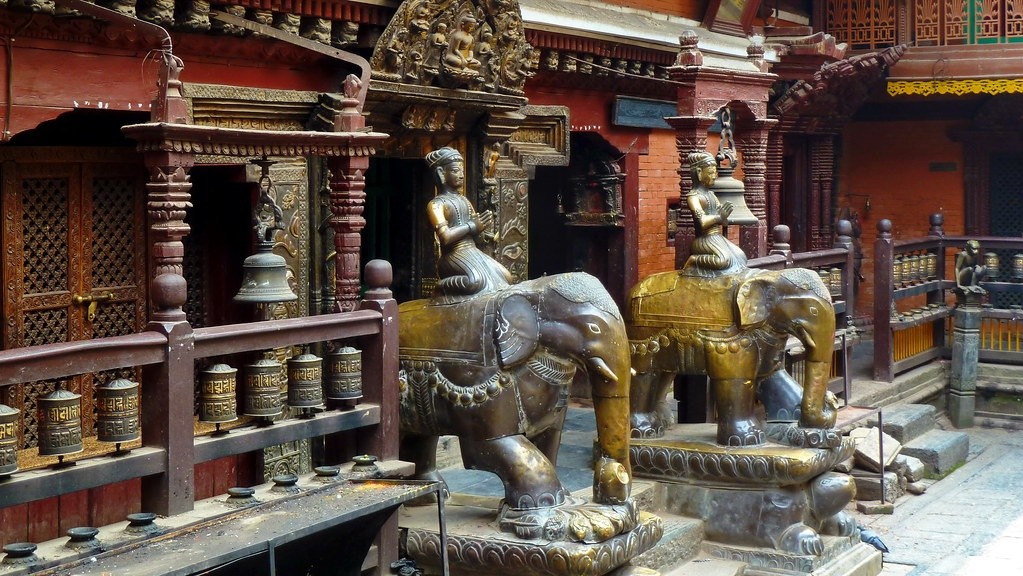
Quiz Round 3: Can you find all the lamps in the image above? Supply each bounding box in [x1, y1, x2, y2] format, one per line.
[846, 193, 871, 211]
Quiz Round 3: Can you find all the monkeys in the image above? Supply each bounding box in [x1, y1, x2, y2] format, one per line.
[953, 240, 992, 295]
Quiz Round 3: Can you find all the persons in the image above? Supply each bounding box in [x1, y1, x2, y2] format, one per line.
[425, 147, 512, 297]
[955, 239, 987, 293]
[686, 152, 746, 269]
[391, 7, 495, 78]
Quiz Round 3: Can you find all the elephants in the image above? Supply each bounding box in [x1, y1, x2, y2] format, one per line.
[622, 261, 837, 446]
[378, 272, 631, 508]
